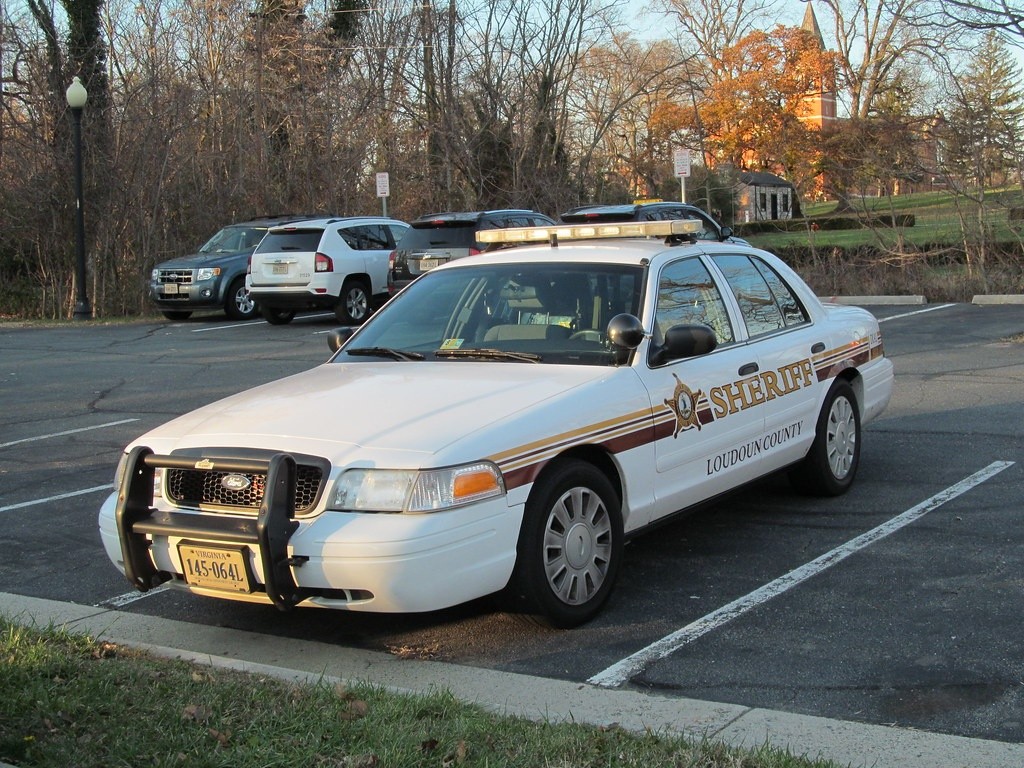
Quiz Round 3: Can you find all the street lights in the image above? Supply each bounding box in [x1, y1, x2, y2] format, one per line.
[66, 76, 93, 321]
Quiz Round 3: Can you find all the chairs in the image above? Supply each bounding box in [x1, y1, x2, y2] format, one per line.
[485, 271, 577, 343]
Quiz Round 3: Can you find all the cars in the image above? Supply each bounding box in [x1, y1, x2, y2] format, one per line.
[99, 219, 894, 630]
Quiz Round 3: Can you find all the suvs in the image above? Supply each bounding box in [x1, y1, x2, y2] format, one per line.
[560, 202, 751, 246]
[245, 216, 410, 325]
[150, 214, 319, 321]
[387, 209, 558, 296]
